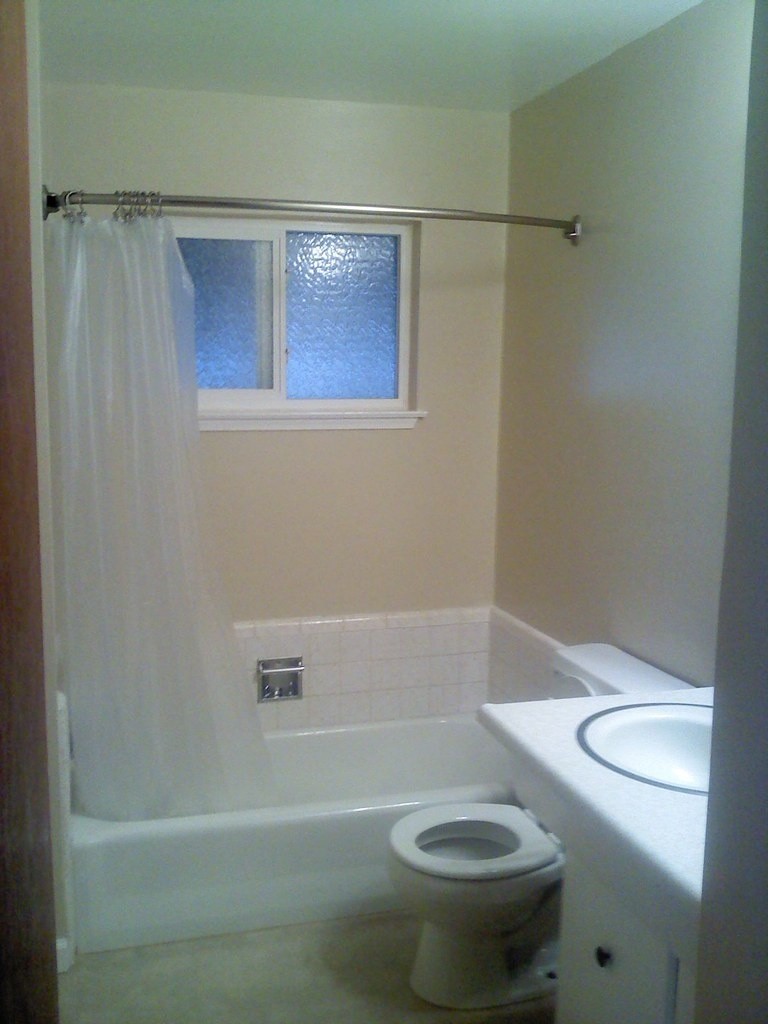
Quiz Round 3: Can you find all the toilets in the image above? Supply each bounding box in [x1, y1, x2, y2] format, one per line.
[387, 641, 701, 1011]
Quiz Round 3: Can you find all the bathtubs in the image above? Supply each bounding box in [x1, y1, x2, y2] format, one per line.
[71, 710, 523, 957]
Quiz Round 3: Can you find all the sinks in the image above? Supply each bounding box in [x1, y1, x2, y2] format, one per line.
[574, 700, 714, 797]
